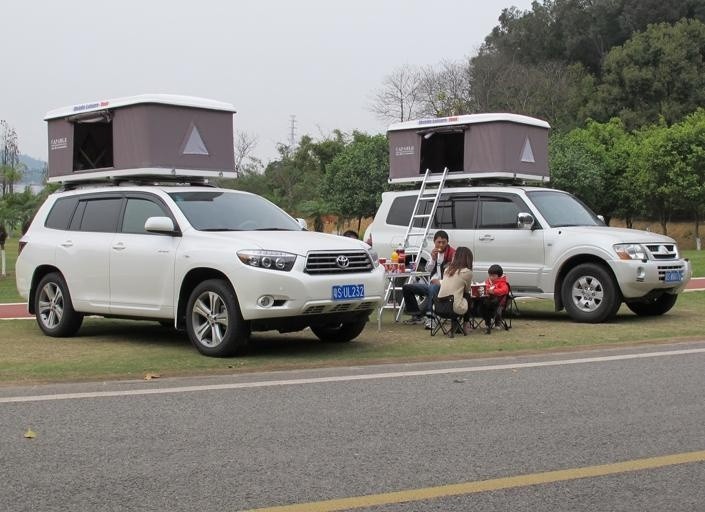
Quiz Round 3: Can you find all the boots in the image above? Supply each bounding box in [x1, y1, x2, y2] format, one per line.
[463, 321, 473, 332]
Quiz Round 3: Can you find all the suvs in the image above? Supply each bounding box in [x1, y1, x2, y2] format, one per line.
[15, 177, 387, 357]
[363, 186, 691, 322]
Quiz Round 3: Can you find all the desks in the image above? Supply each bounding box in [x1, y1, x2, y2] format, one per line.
[383, 271, 431, 323]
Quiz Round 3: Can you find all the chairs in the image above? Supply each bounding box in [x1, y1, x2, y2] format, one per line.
[430, 282, 517, 338]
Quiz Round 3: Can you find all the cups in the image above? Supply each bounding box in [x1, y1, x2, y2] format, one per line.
[383, 263, 405, 273]
[409, 262, 416, 271]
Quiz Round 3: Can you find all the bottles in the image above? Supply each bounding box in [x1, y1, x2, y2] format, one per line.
[391, 242, 405, 263]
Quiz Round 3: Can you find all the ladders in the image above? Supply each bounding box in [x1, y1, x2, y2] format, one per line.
[376, 166, 449, 322]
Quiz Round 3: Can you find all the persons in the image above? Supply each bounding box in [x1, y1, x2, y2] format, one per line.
[400, 230, 456, 329]
[342, 230, 358, 240]
[431, 246, 475, 335]
[477, 264, 509, 333]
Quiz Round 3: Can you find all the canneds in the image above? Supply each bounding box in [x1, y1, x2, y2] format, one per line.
[378, 258, 405, 275]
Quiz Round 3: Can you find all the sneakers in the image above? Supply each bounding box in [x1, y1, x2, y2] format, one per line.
[404, 316, 425, 324]
[485, 321, 504, 330]
[426, 319, 435, 329]
